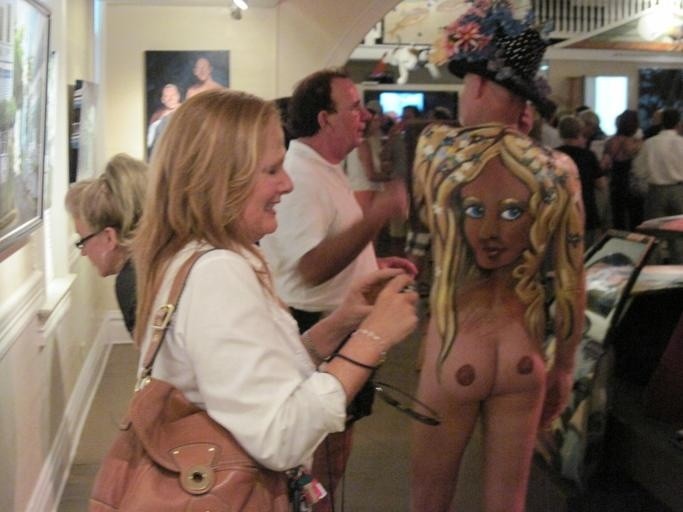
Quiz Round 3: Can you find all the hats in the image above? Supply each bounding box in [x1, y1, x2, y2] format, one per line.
[448, 22, 545, 100]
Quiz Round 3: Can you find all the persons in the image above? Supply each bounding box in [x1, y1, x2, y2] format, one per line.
[410, 71, 587, 512]
[127, 87, 419, 511]
[183, 56, 225, 102]
[258, 68, 409, 512]
[147, 76, 182, 150]
[339, 90, 682, 300]
[65, 152, 159, 346]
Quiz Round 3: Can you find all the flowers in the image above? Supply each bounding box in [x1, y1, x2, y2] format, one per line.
[427, 0, 532, 66]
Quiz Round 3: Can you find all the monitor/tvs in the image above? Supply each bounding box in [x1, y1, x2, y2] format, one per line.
[380, 92, 424, 117]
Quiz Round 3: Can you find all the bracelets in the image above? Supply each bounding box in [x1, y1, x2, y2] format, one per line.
[352, 327, 391, 365]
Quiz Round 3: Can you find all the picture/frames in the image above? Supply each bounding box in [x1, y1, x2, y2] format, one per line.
[0, 0, 58, 256]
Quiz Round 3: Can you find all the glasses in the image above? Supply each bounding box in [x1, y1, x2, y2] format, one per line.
[370, 382, 440, 426]
[75, 224, 115, 249]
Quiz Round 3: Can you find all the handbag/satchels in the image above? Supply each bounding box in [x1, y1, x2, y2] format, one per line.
[87, 380, 289, 512]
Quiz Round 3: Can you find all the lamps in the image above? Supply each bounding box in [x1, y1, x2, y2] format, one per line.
[228, 0, 248, 21]
